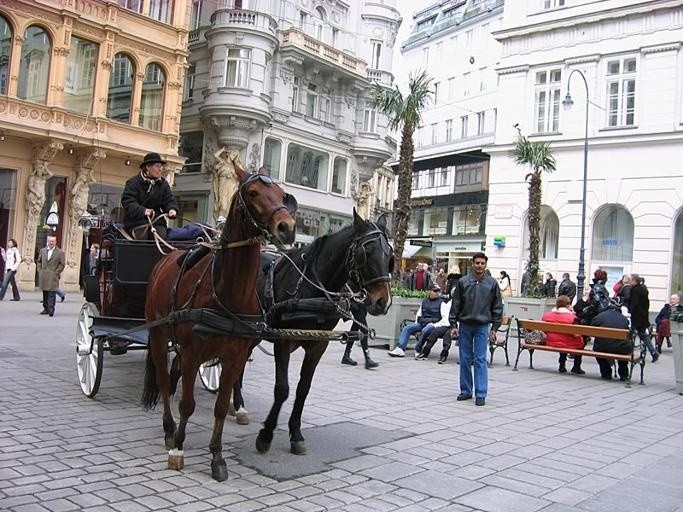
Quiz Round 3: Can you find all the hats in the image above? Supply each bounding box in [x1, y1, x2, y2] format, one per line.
[139, 153, 166, 169]
[430, 284, 441, 291]
[609, 296, 623, 310]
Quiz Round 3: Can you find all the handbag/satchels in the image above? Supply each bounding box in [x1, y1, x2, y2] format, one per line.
[523, 328, 545, 344]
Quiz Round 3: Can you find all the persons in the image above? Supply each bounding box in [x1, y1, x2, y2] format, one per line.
[450, 253, 500, 405]
[35, 234, 65, 317]
[385, 251, 681, 385]
[69, 169, 96, 211]
[0, 239, 22, 301]
[211, 146, 246, 224]
[101, 207, 124, 247]
[89, 242, 99, 276]
[24, 162, 53, 204]
[119, 152, 179, 242]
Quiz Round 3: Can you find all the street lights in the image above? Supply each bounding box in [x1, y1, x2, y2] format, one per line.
[561, 67, 590, 305]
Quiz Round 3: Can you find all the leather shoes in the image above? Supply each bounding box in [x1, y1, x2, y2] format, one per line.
[40, 311, 48, 314]
[49, 312, 54, 316]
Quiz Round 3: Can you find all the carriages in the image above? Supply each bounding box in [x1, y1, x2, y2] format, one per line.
[76, 157, 395, 483]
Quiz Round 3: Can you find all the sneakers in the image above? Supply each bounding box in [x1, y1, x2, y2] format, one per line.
[388, 348, 447, 364]
[652, 351, 659, 363]
[457, 393, 472, 400]
[572, 367, 585, 374]
[559, 362, 566, 372]
[476, 398, 485, 406]
[667, 341, 672, 347]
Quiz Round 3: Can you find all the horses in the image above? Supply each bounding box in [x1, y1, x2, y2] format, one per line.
[228, 206, 393, 455]
[143, 158, 295, 482]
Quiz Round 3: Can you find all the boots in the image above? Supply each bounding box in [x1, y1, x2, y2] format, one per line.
[365, 350, 378, 369]
[342, 349, 358, 366]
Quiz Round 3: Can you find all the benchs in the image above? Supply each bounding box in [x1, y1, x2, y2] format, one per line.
[511, 317, 646, 388]
[399, 315, 513, 367]
[108, 238, 190, 307]
